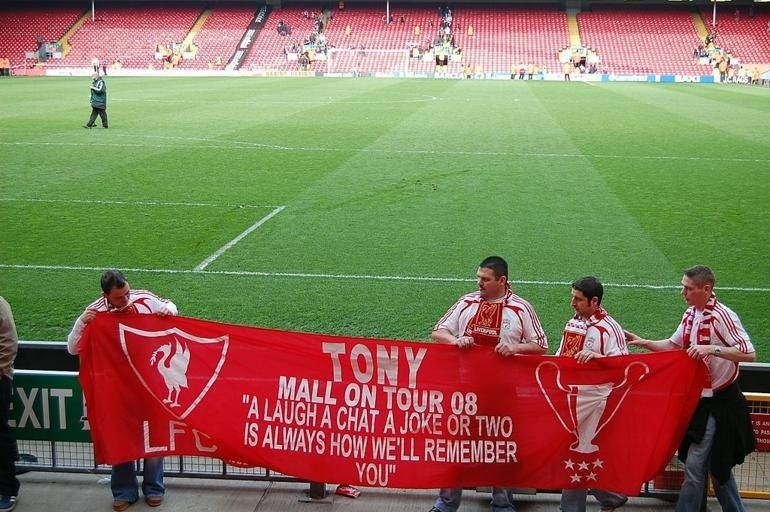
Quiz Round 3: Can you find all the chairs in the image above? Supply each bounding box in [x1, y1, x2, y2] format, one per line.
[0, 6, 770, 77]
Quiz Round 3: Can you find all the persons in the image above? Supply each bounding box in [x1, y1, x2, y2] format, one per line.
[84, 72, 108, 128]
[622, 266, 757, 512]
[694, 28, 761, 84]
[276, 6, 484, 81]
[0, 295, 20, 512]
[512, 43, 608, 81]
[150, 40, 221, 70]
[66, 269, 179, 510]
[0, 33, 71, 76]
[428, 256, 547, 512]
[92, 55, 100, 74]
[103, 61, 107, 75]
[554, 276, 628, 511]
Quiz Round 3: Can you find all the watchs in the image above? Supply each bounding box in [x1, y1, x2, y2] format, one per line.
[713, 344, 721, 357]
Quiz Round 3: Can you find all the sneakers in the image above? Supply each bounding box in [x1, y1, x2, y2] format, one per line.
[146, 496, 163, 506]
[1, 494, 18, 512]
[113, 500, 129, 511]
